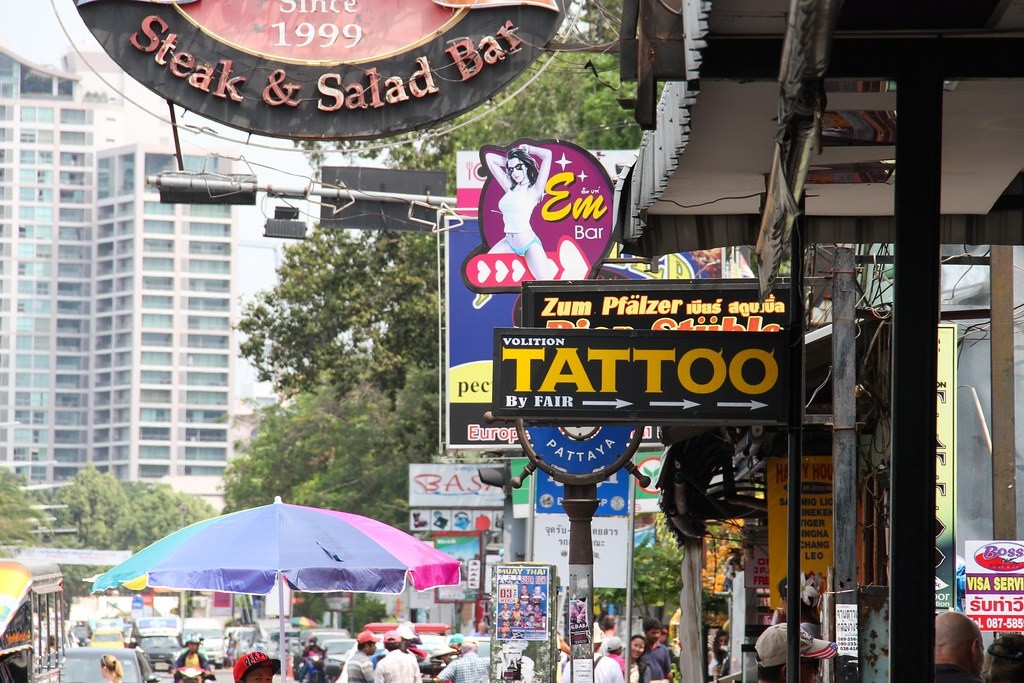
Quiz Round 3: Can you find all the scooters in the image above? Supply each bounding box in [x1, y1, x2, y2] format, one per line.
[174, 667, 214, 682]
[300, 646, 328, 683]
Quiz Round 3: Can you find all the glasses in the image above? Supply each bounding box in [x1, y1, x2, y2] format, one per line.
[509, 163, 524, 173]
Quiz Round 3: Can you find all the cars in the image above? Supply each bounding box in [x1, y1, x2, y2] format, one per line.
[305, 632, 346, 659]
[59, 648, 164, 682]
[225, 625, 301, 654]
[131, 616, 181, 638]
[321, 639, 356, 672]
[128, 634, 186, 673]
[122, 625, 133, 640]
[84, 630, 130, 649]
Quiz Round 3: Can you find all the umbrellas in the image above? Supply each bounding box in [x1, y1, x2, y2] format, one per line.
[80, 493, 463, 683]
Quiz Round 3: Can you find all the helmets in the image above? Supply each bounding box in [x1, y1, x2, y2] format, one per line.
[185, 635, 201, 646]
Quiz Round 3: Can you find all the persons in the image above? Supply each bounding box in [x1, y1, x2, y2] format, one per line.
[299, 637, 326, 682]
[99, 654, 124, 683]
[497, 584, 547, 638]
[753, 571, 839, 682]
[559, 614, 674, 683]
[232, 651, 281, 683]
[980, 631, 1023, 682]
[171, 635, 215, 682]
[346, 621, 489, 683]
[936, 613, 984, 682]
[708, 629, 731, 677]
[471, 143, 560, 308]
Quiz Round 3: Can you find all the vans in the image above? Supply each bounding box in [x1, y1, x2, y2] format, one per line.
[181, 618, 225, 669]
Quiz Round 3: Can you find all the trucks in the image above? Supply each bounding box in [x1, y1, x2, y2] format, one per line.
[0, 557, 65, 683]
[70, 618, 125, 646]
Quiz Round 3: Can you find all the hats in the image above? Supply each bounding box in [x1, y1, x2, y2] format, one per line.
[383, 630, 402, 643]
[755, 621, 838, 668]
[594, 622, 605, 643]
[232, 651, 281, 683]
[397, 625, 418, 640]
[607, 636, 626, 651]
[356, 629, 381, 643]
